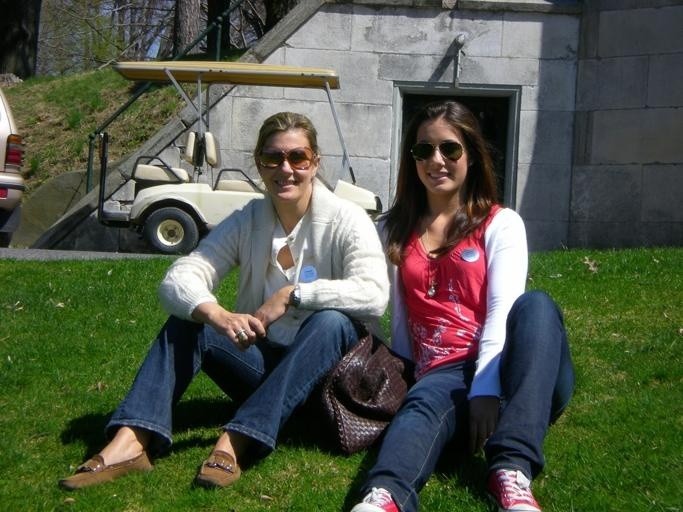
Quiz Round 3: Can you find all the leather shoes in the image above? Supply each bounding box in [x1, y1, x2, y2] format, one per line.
[58, 451, 153, 490]
[196, 449, 242, 487]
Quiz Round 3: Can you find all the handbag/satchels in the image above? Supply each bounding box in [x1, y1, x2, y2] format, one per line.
[323, 332, 408, 455]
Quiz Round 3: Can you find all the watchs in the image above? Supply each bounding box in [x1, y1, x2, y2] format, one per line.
[290, 285, 300, 307]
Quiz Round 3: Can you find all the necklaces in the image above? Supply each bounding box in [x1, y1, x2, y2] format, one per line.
[426, 214, 439, 298]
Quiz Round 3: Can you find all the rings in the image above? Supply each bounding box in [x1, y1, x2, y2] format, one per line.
[237, 329, 246, 340]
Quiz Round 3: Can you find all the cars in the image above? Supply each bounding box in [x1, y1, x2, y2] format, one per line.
[0, 86, 25, 247]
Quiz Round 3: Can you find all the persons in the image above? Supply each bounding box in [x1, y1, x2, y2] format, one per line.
[59, 112, 390, 491]
[350, 102, 573, 512]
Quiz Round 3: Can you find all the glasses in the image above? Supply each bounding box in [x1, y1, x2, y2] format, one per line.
[255, 146, 314, 170]
[411, 142, 465, 161]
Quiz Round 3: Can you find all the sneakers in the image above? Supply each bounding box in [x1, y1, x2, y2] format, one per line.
[484, 469, 541, 512]
[351, 488, 399, 512]
